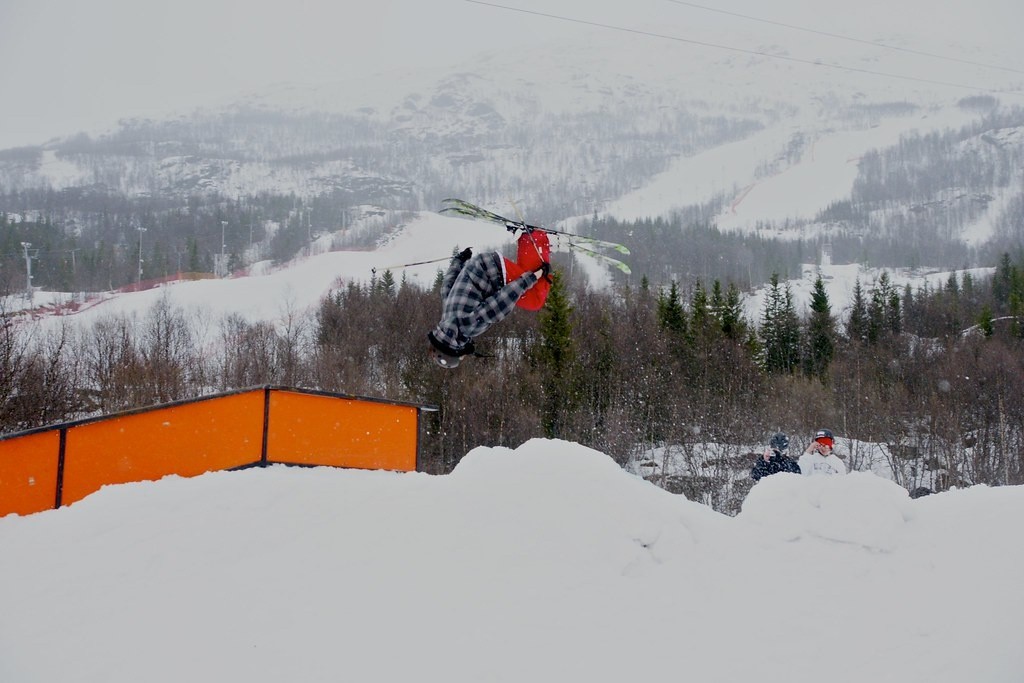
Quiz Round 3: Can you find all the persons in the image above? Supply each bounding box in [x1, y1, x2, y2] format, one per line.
[797, 429, 847, 473]
[426, 229, 554, 369]
[751, 434, 801, 479]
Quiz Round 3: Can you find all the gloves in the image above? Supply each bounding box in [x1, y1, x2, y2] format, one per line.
[460, 248, 471, 260]
[539, 262, 553, 284]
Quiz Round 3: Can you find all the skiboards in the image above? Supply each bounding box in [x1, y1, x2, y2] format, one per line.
[437, 196, 634, 277]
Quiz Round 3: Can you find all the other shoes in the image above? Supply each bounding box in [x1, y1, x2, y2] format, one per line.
[504, 221, 518, 234]
[519, 225, 532, 234]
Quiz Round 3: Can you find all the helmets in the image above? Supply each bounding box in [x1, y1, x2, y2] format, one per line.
[769, 433, 789, 450]
[430, 344, 460, 369]
[814, 429, 834, 441]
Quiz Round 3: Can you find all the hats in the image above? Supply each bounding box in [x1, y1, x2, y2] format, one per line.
[816, 437, 833, 450]
